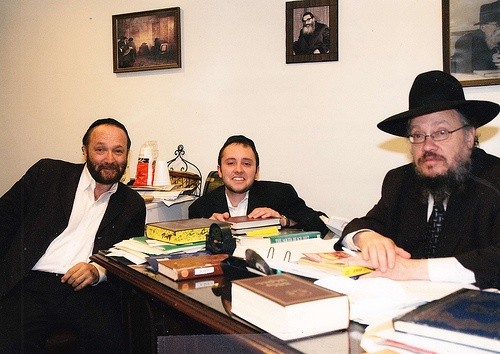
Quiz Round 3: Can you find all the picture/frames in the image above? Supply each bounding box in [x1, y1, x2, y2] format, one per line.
[112, 6, 181, 73]
[442, 0, 500, 87]
[286, 0, 338, 64]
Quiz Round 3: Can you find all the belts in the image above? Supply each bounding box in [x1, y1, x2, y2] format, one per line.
[31, 271, 64, 276]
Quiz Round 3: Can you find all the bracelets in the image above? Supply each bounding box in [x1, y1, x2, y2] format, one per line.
[282, 214, 290, 227]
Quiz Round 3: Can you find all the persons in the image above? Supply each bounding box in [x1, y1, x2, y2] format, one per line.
[333, 70, 500, 292]
[451, 0, 500, 74]
[0, 117, 146, 354]
[187, 135, 329, 240]
[292, 12, 330, 54]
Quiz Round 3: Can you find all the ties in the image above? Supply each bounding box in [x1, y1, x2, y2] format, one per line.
[423, 192, 447, 259]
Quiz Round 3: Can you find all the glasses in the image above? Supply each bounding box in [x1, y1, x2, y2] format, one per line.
[302, 18, 311, 23]
[409, 125, 467, 144]
[484, 23, 500, 27]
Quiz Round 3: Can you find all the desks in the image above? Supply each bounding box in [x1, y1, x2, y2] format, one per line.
[144, 197, 199, 237]
[89, 229, 369, 354]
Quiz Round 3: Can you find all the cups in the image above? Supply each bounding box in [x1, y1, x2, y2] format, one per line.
[153, 160, 170, 186]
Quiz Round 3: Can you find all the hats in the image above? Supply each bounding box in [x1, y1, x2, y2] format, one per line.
[473, 0, 500, 26]
[377, 70, 500, 139]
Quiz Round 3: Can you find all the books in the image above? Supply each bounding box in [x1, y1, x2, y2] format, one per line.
[375, 287, 500, 354]
[224, 217, 321, 244]
[472, 69, 500, 77]
[128, 184, 193, 200]
[288, 251, 374, 277]
[144, 215, 234, 245]
[228, 274, 351, 342]
[145, 253, 230, 282]
